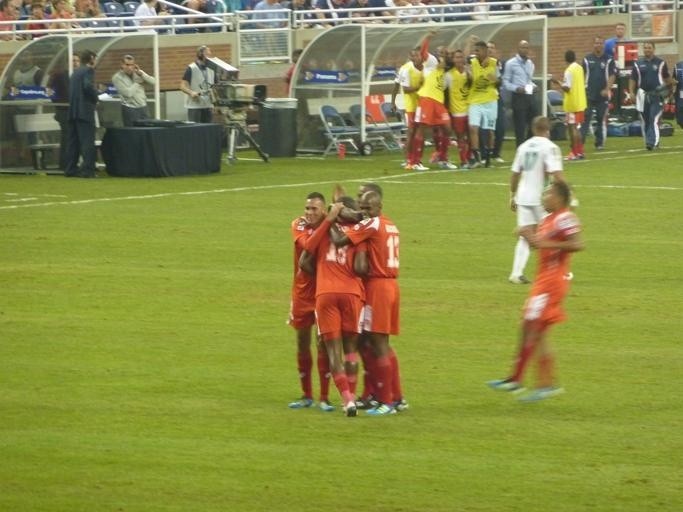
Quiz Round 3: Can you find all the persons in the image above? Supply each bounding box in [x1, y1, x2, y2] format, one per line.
[507, 115, 580, 285]
[51, 50, 103, 177]
[509, 1, 661, 19]
[110, 55, 154, 127]
[285, 182, 411, 416]
[484, 181, 586, 401]
[553, 22, 682, 160]
[249, 1, 490, 29]
[390, 28, 538, 171]
[285, 48, 304, 94]
[5, 49, 44, 141]
[179, 45, 215, 123]
[134, 0, 209, 36]
[2, 0, 102, 42]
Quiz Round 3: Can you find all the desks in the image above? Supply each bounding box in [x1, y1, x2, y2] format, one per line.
[99, 122, 224, 178]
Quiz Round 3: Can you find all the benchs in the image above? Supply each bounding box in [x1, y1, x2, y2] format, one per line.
[12, 108, 104, 169]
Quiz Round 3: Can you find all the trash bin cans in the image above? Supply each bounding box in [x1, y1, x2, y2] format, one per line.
[259, 98, 299, 158]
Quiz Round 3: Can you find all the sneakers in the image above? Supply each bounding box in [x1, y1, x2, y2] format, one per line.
[290, 394, 408, 416]
[530, 384, 564, 402]
[487, 378, 525, 395]
[402, 162, 431, 171]
[564, 153, 585, 161]
[439, 161, 457, 170]
[461, 151, 503, 169]
[507, 274, 529, 285]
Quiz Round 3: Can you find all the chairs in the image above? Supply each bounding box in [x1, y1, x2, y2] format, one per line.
[14, 1, 229, 36]
[319, 105, 368, 157]
[349, 104, 401, 153]
[380, 102, 408, 149]
[427, 1, 558, 24]
[545, 90, 567, 128]
